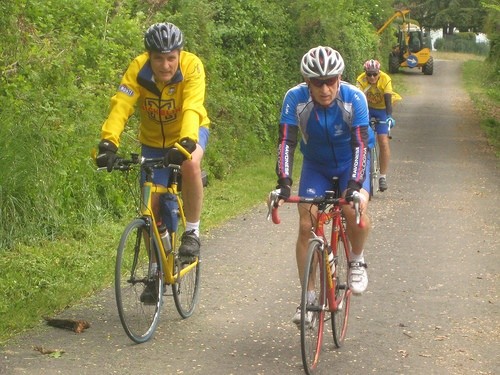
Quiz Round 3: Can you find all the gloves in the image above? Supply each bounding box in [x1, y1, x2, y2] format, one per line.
[385, 116, 395, 128]
[96, 139, 118, 172]
[164, 137, 196, 167]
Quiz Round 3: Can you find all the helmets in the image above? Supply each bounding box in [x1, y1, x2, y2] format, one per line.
[145, 22, 183, 53]
[364, 60, 380, 72]
[301, 46, 344, 78]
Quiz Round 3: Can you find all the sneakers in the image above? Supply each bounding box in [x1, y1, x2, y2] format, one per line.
[348, 259, 369, 294]
[379, 177, 387, 190]
[293, 310, 316, 330]
[179, 229, 200, 255]
[141, 276, 167, 306]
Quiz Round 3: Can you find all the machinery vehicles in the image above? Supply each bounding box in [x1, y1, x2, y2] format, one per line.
[388, 9, 434, 75]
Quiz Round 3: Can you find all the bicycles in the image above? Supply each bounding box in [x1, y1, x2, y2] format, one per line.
[96, 140, 203, 344]
[368, 116, 393, 202]
[265, 189, 366, 375]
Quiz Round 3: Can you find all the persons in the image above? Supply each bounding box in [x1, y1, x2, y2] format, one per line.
[352, 59, 398, 191]
[271, 44, 375, 330]
[93, 23, 210, 304]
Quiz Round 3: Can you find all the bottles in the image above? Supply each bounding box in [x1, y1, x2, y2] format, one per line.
[157, 222, 172, 252]
[328, 245, 335, 275]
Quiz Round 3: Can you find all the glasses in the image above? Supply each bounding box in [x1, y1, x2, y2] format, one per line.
[308, 76, 339, 86]
[366, 72, 378, 77]
